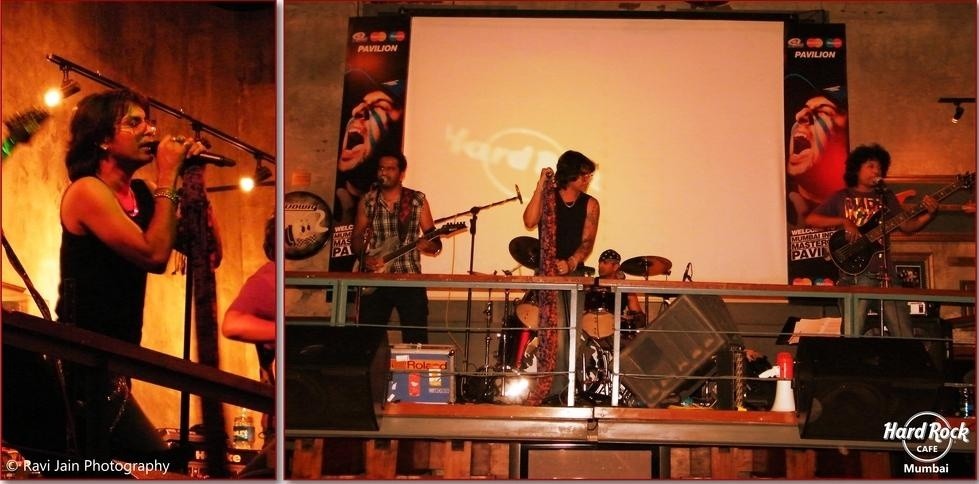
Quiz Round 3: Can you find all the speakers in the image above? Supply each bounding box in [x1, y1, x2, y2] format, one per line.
[285, 327, 392, 431]
[607, 294, 741, 407]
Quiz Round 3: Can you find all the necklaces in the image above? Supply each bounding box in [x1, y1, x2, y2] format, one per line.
[560, 187, 580, 208]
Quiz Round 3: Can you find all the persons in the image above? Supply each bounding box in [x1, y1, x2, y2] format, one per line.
[332, 65, 407, 238]
[509, 149, 600, 277]
[584, 250, 646, 352]
[344, 149, 442, 344]
[788, 83, 848, 257]
[49, 84, 223, 483]
[805, 142, 939, 339]
[221, 211, 276, 463]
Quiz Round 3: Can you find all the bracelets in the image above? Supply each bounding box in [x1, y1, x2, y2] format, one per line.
[153, 187, 182, 211]
[568, 256, 577, 273]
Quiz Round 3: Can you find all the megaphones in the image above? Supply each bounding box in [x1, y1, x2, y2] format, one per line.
[758, 353, 797, 411]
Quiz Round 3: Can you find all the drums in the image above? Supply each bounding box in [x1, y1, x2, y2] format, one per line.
[583, 287, 615, 339]
[514, 290, 539, 333]
[523, 330, 608, 403]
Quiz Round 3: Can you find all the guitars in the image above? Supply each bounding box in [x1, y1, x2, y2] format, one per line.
[829, 171, 976, 276]
[352, 222, 468, 297]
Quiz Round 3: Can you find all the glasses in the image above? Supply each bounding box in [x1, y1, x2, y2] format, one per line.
[112, 118, 158, 137]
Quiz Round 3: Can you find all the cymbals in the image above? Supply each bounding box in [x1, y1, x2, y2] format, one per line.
[509, 236, 539, 270]
[620, 256, 672, 276]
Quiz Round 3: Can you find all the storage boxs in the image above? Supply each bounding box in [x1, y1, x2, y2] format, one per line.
[386, 342, 456, 407]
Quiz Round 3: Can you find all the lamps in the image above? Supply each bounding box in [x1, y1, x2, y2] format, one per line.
[277, 320, 394, 432]
[35, 48, 276, 193]
[935, 97, 977, 123]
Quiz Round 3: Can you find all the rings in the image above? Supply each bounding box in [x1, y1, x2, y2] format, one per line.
[173, 137, 183, 143]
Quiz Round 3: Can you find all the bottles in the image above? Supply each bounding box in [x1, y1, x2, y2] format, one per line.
[231, 405, 254, 444]
[960, 386, 975, 416]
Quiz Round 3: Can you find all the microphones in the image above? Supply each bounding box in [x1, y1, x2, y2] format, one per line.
[544, 168, 554, 178]
[148, 138, 237, 169]
[872, 176, 886, 190]
[372, 178, 383, 189]
